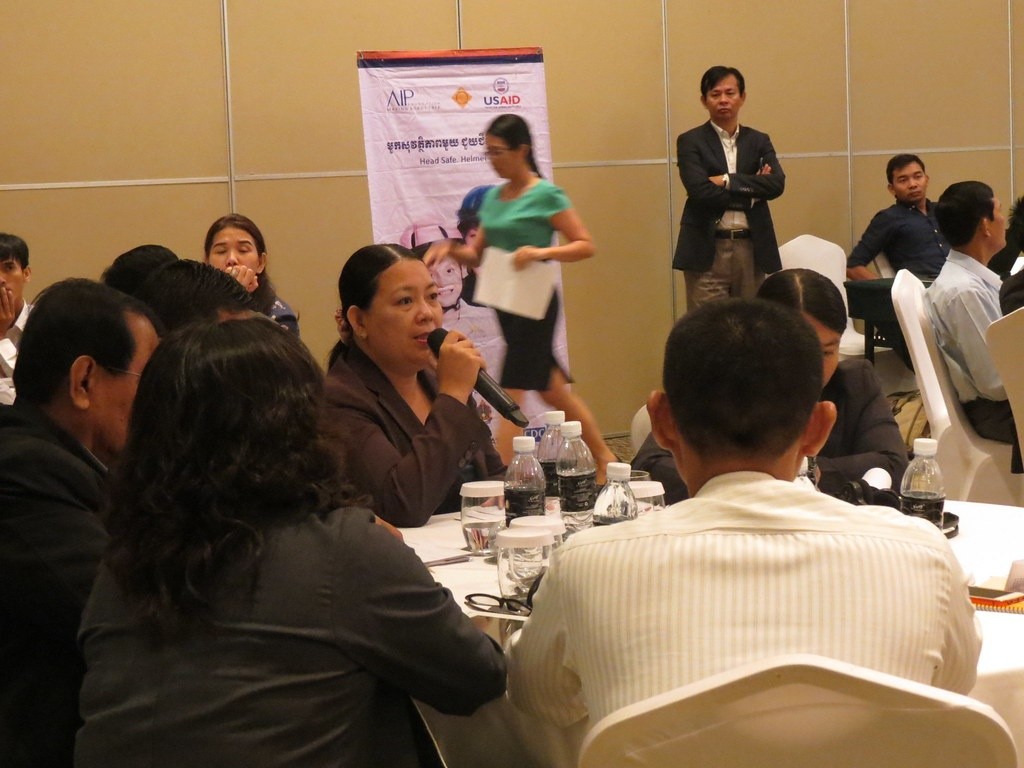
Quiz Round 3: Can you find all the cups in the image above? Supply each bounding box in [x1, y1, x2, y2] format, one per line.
[630, 469, 651, 481]
[496, 529, 554, 607]
[508, 515, 566, 559]
[627, 481, 665, 515]
[459, 480, 506, 552]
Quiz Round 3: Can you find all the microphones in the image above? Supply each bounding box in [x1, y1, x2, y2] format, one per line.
[427, 328, 530, 428]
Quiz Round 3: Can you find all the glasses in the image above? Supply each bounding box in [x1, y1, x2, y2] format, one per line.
[464, 593, 532, 618]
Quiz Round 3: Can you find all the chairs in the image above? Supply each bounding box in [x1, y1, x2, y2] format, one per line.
[630, 234, 1023, 507]
[578, 653, 1018, 768]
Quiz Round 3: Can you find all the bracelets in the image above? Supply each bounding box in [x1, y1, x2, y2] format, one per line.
[723, 174, 727, 189]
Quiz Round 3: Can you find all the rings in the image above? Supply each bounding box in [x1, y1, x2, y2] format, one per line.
[230, 270, 239, 276]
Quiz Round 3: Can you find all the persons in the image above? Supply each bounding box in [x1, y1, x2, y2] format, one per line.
[846, 154, 1024, 474]
[0, 215, 301, 768]
[669, 65, 787, 314]
[631, 267, 909, 506]
[422, 113, 623, 496]
[400, 185, 508, 483]
[502, 301, 982, 768]
[72, 317, 506, 768]
[312, 243, 508, 530]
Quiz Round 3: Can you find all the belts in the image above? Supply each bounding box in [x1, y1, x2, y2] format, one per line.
[715, 228, 753, 241]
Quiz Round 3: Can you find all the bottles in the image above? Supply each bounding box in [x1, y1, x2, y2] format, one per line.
[793, 457, 817, 491]
[538, 411, 566, 516]
[900, 436, 946, 536]
[556, 421, 598, 541]
[593, 462, 637, 526]
[504, 436, 546, 529]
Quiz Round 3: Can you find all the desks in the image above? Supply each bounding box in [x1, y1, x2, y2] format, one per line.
[398, 494, 1023, 768]
[844, 278, 935, 365]
